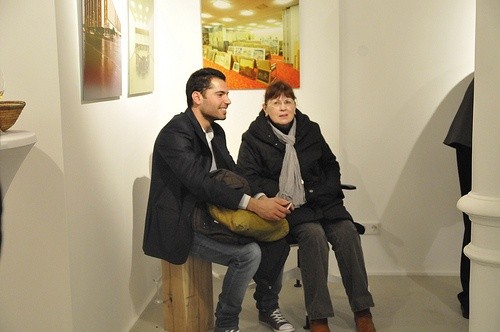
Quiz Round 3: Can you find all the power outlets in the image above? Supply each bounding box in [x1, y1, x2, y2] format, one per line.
[361, 222, 380, 235]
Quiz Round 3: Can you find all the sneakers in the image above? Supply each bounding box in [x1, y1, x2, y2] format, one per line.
[259, 309, 296, 332]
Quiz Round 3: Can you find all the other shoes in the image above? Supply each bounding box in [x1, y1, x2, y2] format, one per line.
[354, 311, 376, 332]
[309, 319, 330, 332]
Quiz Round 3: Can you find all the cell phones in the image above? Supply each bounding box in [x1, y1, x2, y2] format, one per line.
[285, 203, 292, 209]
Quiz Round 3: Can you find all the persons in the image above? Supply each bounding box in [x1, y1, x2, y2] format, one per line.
[143, 68, 295, 332]
[444, 77, 474, 319]
[237, 81, 376, 332]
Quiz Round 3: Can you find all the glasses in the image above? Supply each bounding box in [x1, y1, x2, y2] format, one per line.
[267, 99, 295, 106]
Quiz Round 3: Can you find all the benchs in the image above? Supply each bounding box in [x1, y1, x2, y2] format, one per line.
[159, 254, 215, 332]
